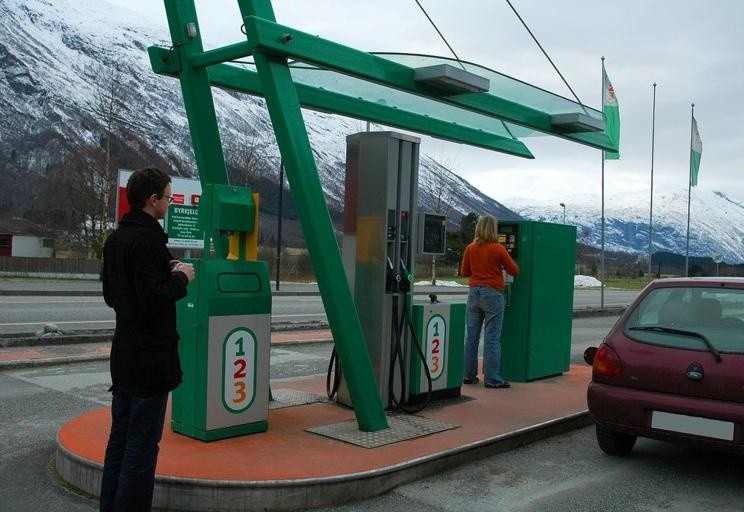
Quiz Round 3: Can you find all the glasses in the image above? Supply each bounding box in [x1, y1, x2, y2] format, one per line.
[158, 192, 174, 203]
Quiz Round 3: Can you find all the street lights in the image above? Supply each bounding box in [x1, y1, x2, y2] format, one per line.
[559, 203, 566, 224]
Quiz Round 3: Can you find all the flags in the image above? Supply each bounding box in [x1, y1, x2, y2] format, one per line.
[601, 67, 621, 160]
[689, 116, 702, 187]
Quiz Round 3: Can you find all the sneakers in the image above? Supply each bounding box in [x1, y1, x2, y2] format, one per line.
[483, 380, 512, 389]
[462, 376, 480, 385]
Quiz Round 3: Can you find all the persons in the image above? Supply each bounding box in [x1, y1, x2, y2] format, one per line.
[98, 167, 197, 512]
[459, 213, 519, 388]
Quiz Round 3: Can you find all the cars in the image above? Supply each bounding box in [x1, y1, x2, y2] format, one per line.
[580, 275, 743, 461]
